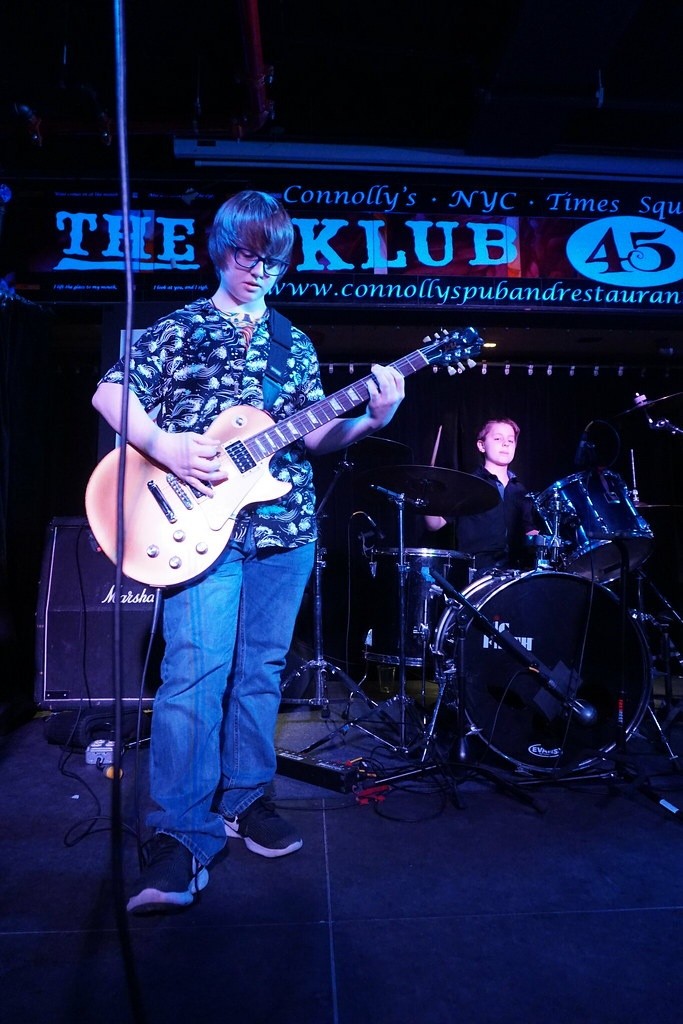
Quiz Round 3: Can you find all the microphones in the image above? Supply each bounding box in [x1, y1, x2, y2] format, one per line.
[363, 513, 390, 553]
[574, 433, 588, 475]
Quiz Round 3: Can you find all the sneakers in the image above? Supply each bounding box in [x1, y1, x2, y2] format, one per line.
[221, 792, 303, 858]
[126, 833, 209, 911]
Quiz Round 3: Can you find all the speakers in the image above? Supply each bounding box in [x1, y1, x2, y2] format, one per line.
[36, 515, 329, 711]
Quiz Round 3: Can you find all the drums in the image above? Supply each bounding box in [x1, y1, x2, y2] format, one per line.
[535, 466, 655, 579]
[418, 562, 654, 777]
[354, 543, 475, 665]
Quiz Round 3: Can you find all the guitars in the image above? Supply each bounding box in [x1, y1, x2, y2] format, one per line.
[84, 328, 481, 579]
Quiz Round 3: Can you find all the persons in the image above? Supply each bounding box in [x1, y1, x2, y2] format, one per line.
[421, 417, 542, 583]
[87, 184, 404, 918]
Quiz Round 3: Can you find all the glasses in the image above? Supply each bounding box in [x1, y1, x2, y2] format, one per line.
[228, 239, 288, 276]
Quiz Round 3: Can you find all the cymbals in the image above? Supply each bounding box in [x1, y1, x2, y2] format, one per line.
[358, 463, 502, 516]
[328, 429, 420, 462]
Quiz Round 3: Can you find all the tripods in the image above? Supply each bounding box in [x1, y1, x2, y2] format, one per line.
[297, 484, 466, 809]
[277, 465, 394, 719]
